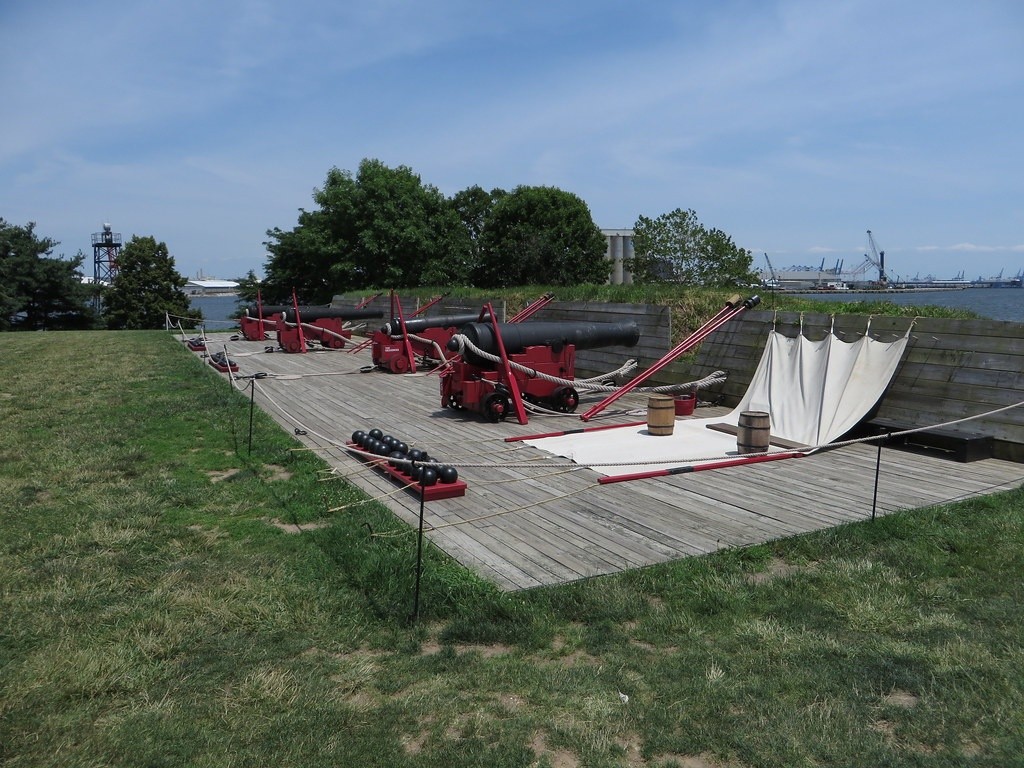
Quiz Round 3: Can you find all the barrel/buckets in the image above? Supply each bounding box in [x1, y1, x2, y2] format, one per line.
[737, 411, 770, 456]
[648, 397, 675, 436]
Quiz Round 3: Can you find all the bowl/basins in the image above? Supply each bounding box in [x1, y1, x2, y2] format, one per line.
[672, 393, 695, 415]
